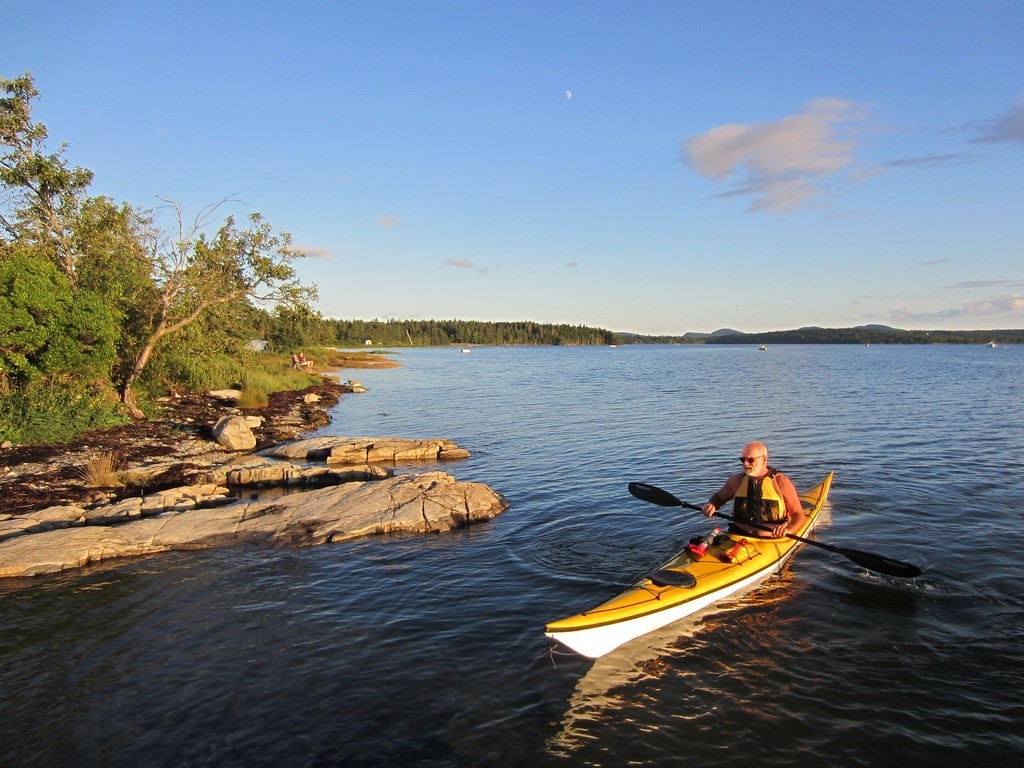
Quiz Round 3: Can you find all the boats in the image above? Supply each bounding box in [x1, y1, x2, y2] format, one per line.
[988, 342, 995, 348]
[758, 346, 768, 350]
[461, 349, 471, 352]
[543, 472, 835, 658]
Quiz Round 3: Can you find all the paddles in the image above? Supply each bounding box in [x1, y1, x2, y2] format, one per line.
[628, 480, 922, 578]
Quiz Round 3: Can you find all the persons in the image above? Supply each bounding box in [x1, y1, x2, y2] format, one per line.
[298, 352, 314, 371]
[292, 353, 302, 370]
[700, 440, 807, 539]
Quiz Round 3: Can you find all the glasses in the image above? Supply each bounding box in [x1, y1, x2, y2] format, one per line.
[739, 455, 762, 463]
